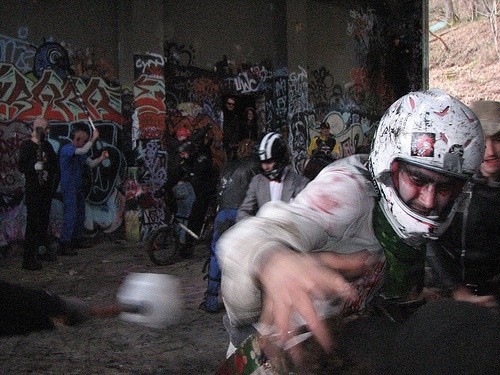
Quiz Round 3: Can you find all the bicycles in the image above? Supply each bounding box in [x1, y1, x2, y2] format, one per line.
[147, 188, 230, 267]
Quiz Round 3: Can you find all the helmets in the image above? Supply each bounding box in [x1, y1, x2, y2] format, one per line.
[256, 132, 288, 180]
[368, 89, 485, 250]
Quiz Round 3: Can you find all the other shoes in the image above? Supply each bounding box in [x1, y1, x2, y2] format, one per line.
[71, 239, 90, 249]
[178, 246, 194, 259]
[56, 248, 77, 257]
[21, 261, 43, 271]
[164, 204, 176, 224]
[38, 253, 57, 262]
[198, 301, 219, 315]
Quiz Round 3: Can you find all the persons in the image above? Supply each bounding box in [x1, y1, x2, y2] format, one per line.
[155, 129, 221, 260]
[17, 117, 59, 271]
[222, 96, 257, 144]
[235, 132, 311, 225]
[217, 134, 261, 215]
[214, 87, 500, 375]
[424, 100, 500, 307]
[57, 126, 109, 255]
[307, 121, 344, 160]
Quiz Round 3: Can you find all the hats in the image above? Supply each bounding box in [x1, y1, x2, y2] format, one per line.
[320, 122, 330, 129]
[470, 99, 500, 137]
